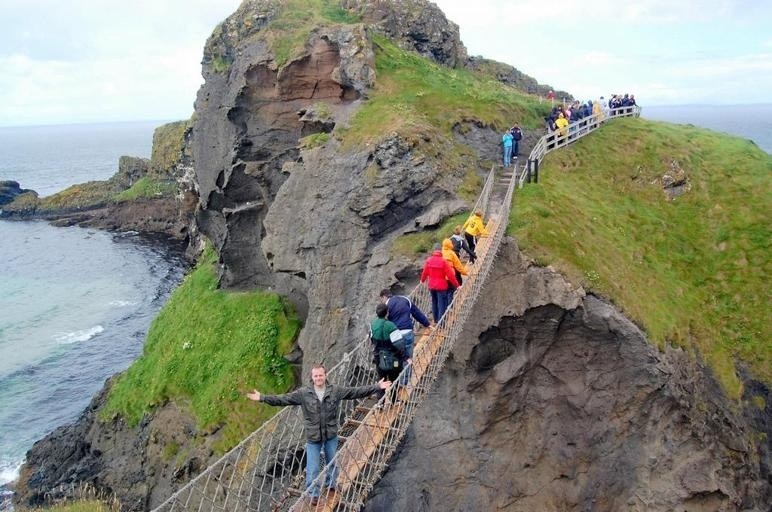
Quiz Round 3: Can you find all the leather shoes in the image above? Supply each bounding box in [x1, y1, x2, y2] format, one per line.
[332, 485, 342, 492]
[312, 496, 318, 505]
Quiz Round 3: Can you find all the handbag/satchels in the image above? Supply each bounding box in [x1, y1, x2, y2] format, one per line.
[378, 350, 404, 374]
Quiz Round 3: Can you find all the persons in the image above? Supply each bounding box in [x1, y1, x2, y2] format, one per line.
[245, 366, 393, 505]
[370, 304, 413, 409]
[380, 283, 434, 385]
[420, 242, 462, 328]
[441, 237, 471, 306]
[449, 226, 479, 286]
[502, 88, 637, 169]
[459, 208, 489, 264]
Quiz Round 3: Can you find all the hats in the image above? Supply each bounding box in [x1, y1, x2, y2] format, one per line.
[475, 210, 482, 216]
[434, 244, 441, 250]
[455, 229, 460, 234]
